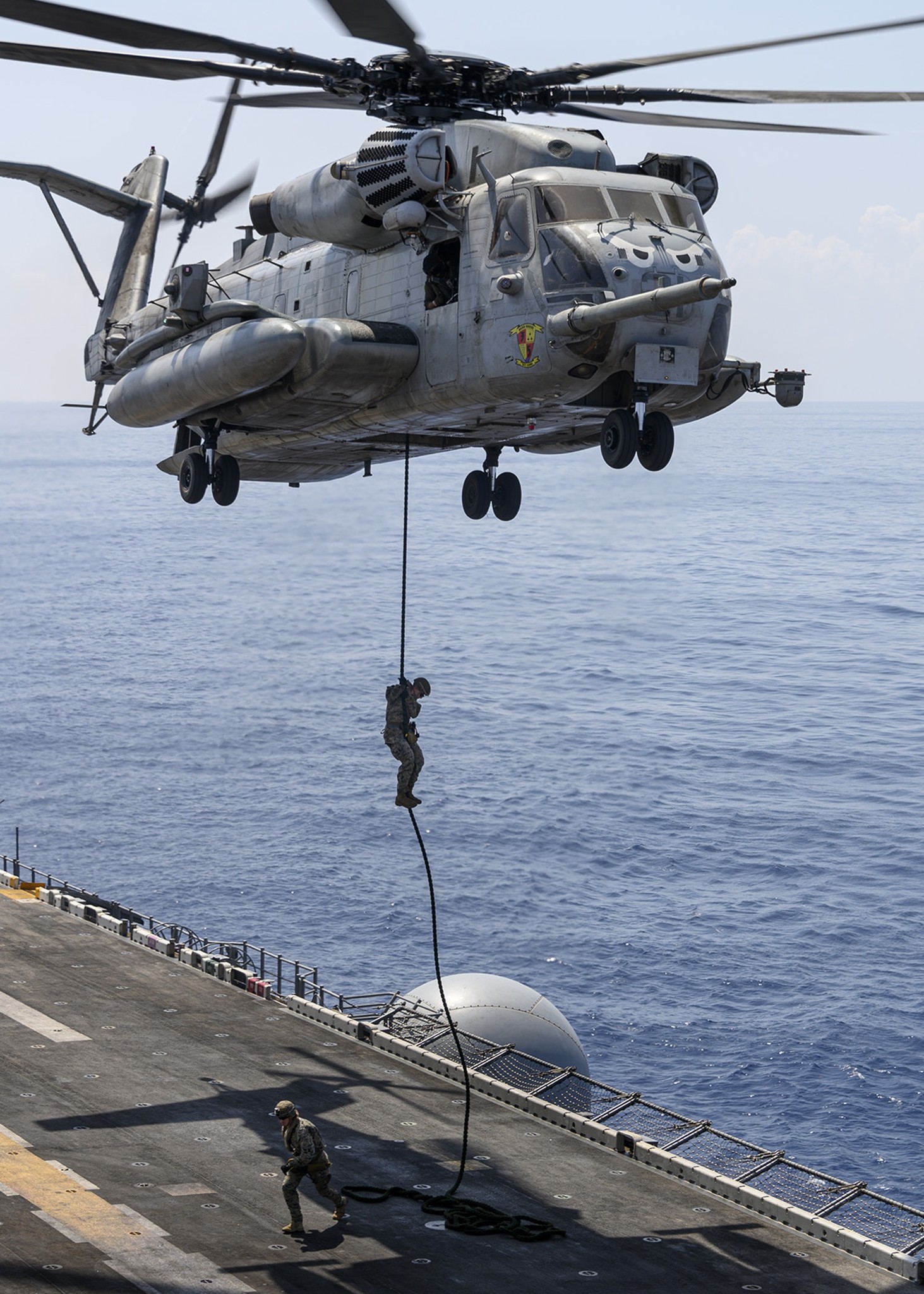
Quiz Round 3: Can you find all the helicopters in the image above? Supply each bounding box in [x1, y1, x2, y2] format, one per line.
[0, 0, 924, 518]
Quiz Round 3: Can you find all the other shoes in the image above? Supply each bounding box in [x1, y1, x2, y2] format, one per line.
[332, 1196, 349, 1220]
[281, 1220, 304, 1232]
[395, 794, 422, 808]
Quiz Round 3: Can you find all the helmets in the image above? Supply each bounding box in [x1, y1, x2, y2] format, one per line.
[413, 676, 431, 696]
[275, 1100, 295, 1120]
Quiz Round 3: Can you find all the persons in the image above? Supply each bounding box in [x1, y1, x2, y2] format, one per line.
[272, 1099, 348, 1233]
[383, 675, 432, 808]
[420, 249, 459, 310]
